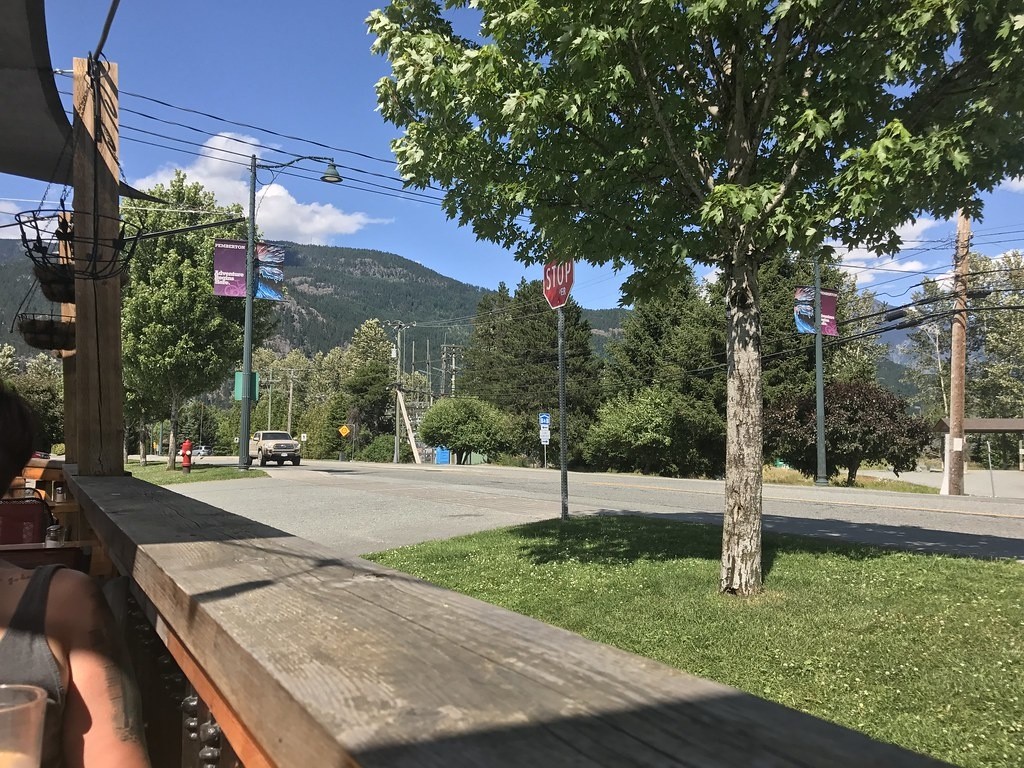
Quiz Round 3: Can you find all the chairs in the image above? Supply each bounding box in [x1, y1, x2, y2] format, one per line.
[0, 487, 54, 545]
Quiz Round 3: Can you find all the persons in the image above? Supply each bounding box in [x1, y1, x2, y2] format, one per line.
[0, 380, 149, 768]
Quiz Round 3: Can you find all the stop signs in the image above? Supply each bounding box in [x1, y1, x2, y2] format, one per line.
[543, 255, 574, 311]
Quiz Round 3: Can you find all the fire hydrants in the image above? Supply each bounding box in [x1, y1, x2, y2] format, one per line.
[178, 439, 192, 474]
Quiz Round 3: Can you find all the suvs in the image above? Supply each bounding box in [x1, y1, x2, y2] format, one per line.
[249, 431, 301, 467]
[192, 446, 212, 456]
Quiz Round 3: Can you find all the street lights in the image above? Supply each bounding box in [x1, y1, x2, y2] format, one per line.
[238, 155, 343, 474]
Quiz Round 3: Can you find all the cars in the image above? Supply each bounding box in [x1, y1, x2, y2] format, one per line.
[31, 451, 50, 459]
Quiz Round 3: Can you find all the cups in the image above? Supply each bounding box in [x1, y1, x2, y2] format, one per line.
[54, 487, 70, 505]
[0, 683, 47, 768]
[46, 525, 66, 548]
